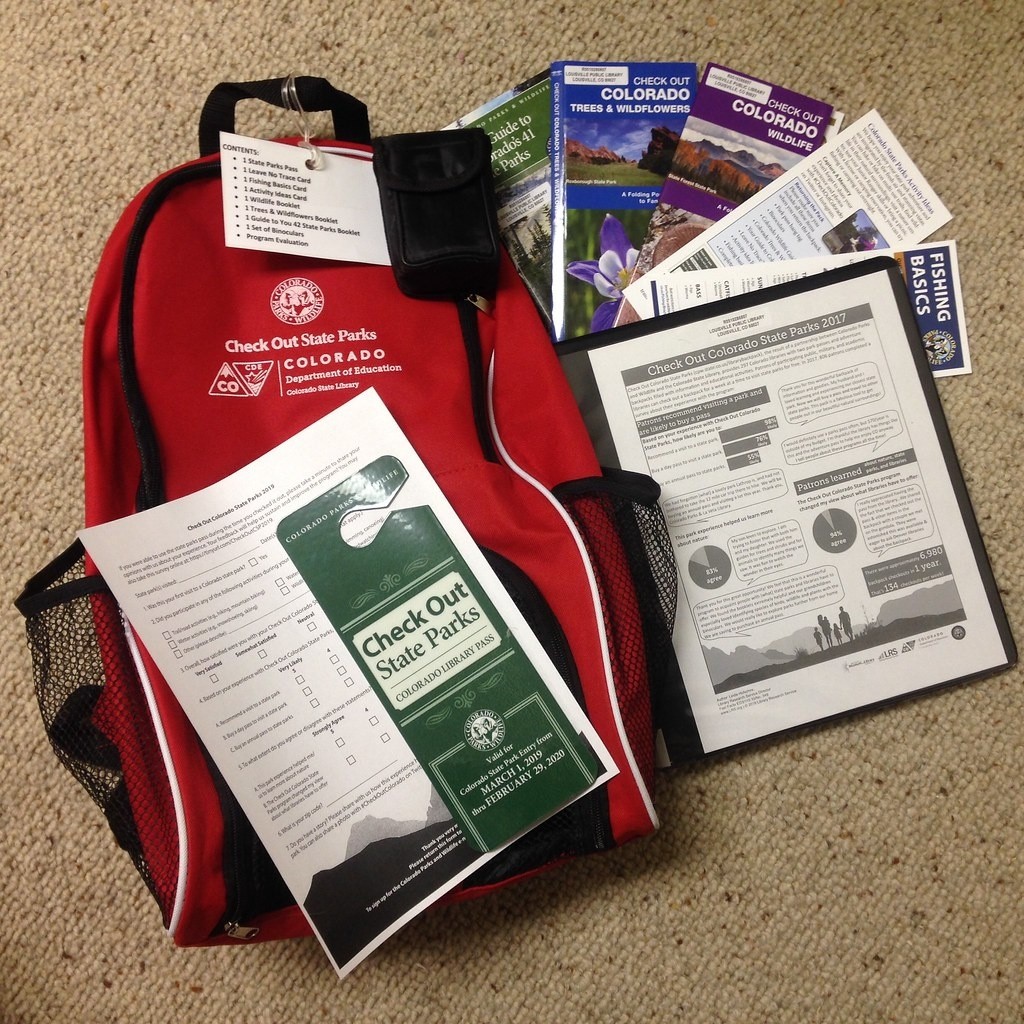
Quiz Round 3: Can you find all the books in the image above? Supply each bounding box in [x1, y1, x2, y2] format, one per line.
[437, 60, 699, 343]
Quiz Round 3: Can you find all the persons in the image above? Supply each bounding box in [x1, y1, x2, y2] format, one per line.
[813, 606, 854, 652]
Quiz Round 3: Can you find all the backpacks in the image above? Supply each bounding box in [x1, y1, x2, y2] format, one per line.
[12, 75, 676, 948]
